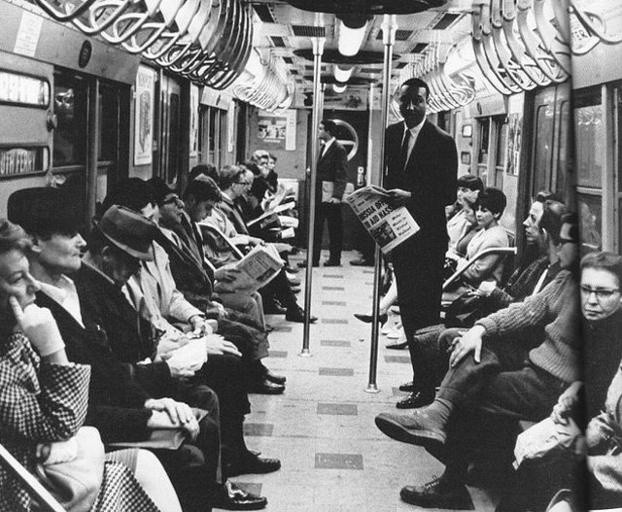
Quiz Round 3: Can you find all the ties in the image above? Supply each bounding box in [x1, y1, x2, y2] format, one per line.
[171, 232, 181, 248]
[513, 258, 551, 302]
[399, 128, 412, 173]
[190, 219, 204, 264]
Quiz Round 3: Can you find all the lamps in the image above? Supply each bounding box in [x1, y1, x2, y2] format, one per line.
[332, 19, 369, 94]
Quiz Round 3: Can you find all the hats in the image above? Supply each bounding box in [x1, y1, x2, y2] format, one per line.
[6, 184, 90, 235]
[91, 203, 157, 262]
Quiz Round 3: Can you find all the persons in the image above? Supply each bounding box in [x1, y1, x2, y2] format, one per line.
[0, 151, 317, 512]
[257, 116, 286, 140]
[386, 77, 457, 409]
[298, 120, 348, 268]
[354, 176, 621, 510]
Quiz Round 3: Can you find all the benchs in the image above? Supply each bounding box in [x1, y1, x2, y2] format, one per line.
[417, 236, 588, 468]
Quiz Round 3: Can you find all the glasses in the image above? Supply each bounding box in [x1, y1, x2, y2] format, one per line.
[580, 283, 622, 302]
[233, 180, 250, 185]
[105, 242, 140, 268]
[159, 195, 180, 206]
[556, 235, 584, 245]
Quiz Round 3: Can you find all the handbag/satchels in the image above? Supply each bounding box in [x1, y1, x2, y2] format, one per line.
[322, 181, 354, 204]
[34, 424, 106, 512]
[445, 290, 493, 327]
[108, 403, 209, 452]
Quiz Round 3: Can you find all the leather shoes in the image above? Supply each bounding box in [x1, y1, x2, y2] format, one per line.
[349, 259, 374, 266]
[401, 475, 474, 512]
[285, 309, 317, 323]
[222, 452, 281, 479]
[397, 383, 435, 410]
[246, 374, 286, 395]
[286, 259, 341, 274]
[375, 412, 447, 448]
[263, 277, 301, 312]
[208, 481, 267, 512]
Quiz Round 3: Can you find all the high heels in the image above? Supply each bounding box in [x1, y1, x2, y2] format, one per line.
[386, 339, 409, 351]
[353, 312, 388, 328]
